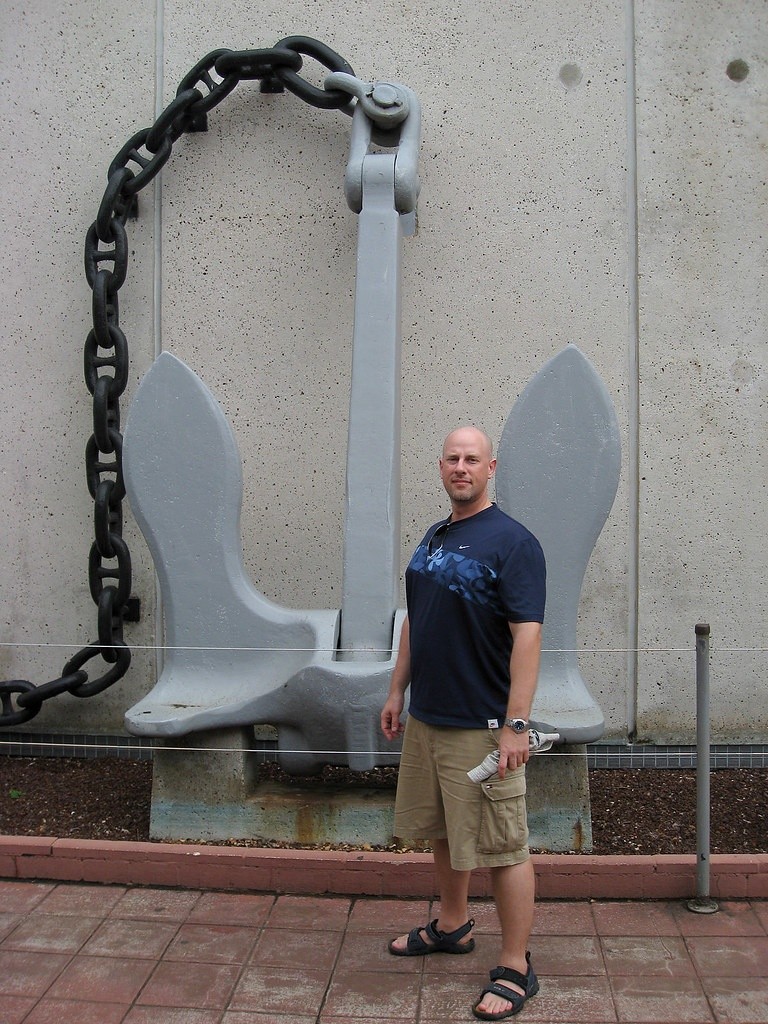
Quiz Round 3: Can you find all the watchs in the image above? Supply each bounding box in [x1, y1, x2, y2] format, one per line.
[504, 718, 530, 733]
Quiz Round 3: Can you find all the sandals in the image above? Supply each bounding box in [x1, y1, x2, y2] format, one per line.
[472, 951, 540, 1021]
[388, 917, 476, 957]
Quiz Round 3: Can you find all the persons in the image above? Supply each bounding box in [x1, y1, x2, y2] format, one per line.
[379, 426, 547, 1022]
